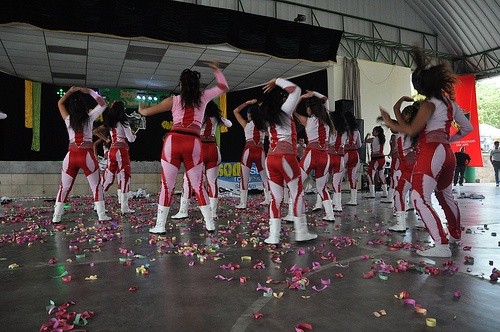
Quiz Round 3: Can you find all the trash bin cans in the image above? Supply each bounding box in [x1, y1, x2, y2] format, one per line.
[465, 167, 476, 183]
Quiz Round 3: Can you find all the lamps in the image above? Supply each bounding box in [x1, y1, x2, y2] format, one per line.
[136, 92, 158, 101]
[293, 14, 306, 23]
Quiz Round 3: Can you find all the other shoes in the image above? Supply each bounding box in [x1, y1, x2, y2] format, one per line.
[416, 245, 452, 257]
[393, 205, 397, 217]
[414, 219, 424, 230]
[449, 234, 461, 244]
[453, 184, 456, 186]
[496, 183, 499, 186]
[459, 184, 463, 186]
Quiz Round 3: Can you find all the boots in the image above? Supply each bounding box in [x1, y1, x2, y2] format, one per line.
[120, 193, 136, 214]
[332, 193, 343, 212]
[197, 204, 216, 233]
[381, 184, 388, 198]
[235, 190, 248, 210]
[380, 189, 395, 203]
[260, 188, 273, 206]
[294, 215, 319, 243]
[312, 194, 322, 212]
[322, 200, 335, 222]
[171, 195, 189, 220]
[149, 204, 171, 235]
[264, 218, 282, 247]
[388, 212, 406, 233]
[346, 189, 358, 206]
[365, 185, 375, 198]
[281, 198, 294, 222]
[51, 202, 66, 224]
[93, 200, 113, 222]
[406, 210, 414, 230]
[117, 189, 122, 204]
[209, 196, 218, 221]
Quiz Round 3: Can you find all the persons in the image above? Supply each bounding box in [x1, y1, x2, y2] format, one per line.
[376, 94, 439, 245]
[364, 126, 389, 198]
[51, 86, 113, 223]
[333, 111, 362, 206]
[251, 78, 318, 244]
[170, 101, 232, 221]
[490, 141, 500, 186]
[295, 137, 308, 160]
[453, 148, 471, 186]
[233, 98, 268, 208]
[310, 112, 350, 212]
[394, 45, 472, 255]
[139, 62, 228, 234]
[280, 90, 335, 221]
[93, 101, 137, 214]
